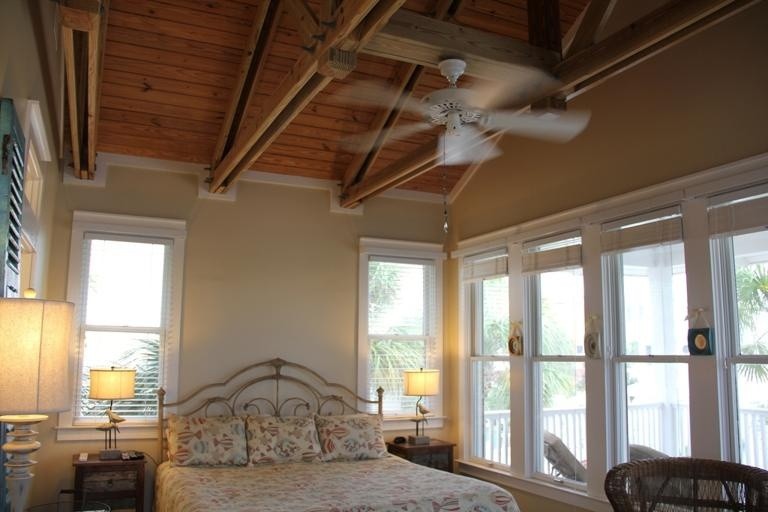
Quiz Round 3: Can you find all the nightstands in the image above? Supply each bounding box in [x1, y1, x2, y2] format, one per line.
[71, 446, 147, 511]
[386, 436, 457, 474]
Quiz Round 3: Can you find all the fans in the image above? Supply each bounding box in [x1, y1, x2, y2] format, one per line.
[327, 59, 594, 174]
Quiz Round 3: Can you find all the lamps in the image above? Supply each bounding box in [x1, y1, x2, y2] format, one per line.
[400, 364, 443, 445]
[1, 282, 77, 512]
[86, 362, 141, 450]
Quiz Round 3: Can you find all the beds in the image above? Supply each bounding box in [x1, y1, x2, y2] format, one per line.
[150, 354, 522, 511]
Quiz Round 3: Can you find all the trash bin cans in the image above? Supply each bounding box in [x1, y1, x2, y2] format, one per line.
[56, 488, 75, 512]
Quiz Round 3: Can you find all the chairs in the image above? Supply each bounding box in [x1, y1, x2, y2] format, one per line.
[544, 429, 668, 482]
[601, 455, 767, 512]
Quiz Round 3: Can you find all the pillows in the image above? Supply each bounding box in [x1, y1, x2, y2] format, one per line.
[165, 411, 249, 470]
[245, 410, 324, 467]
[313, 411, 389, 464]
